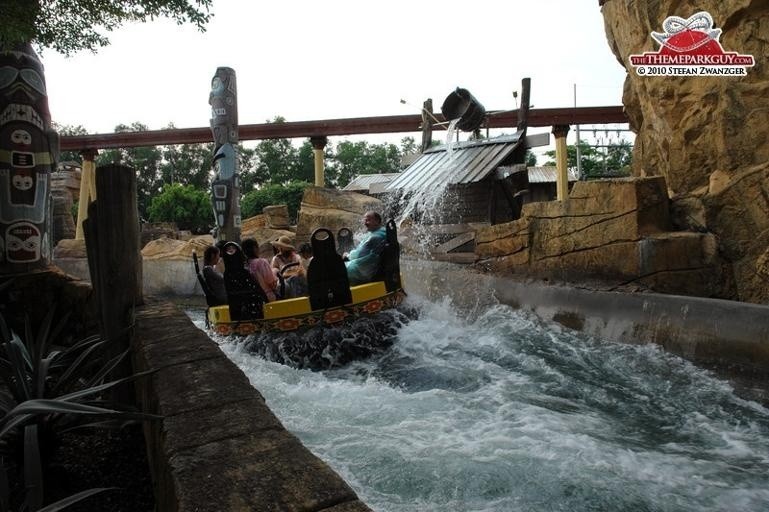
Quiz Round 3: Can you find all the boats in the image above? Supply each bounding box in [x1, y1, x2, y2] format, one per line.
[192, 228, 421, 372]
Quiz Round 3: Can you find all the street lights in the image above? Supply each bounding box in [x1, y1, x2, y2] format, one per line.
[401, 99, 449, 131]
[513, 91, 517, 113]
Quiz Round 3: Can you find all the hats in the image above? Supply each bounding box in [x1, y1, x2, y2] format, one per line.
[270, 235, 295, 252]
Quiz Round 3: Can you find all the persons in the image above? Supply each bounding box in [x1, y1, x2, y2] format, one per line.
[297, 242, 316, 273]
[269, 234, 309, 302]
[212, 239, 230, 280]
[339, 210, 388, 284]
[200, 246, 227, 307]
[236, 239, 280, 303]
